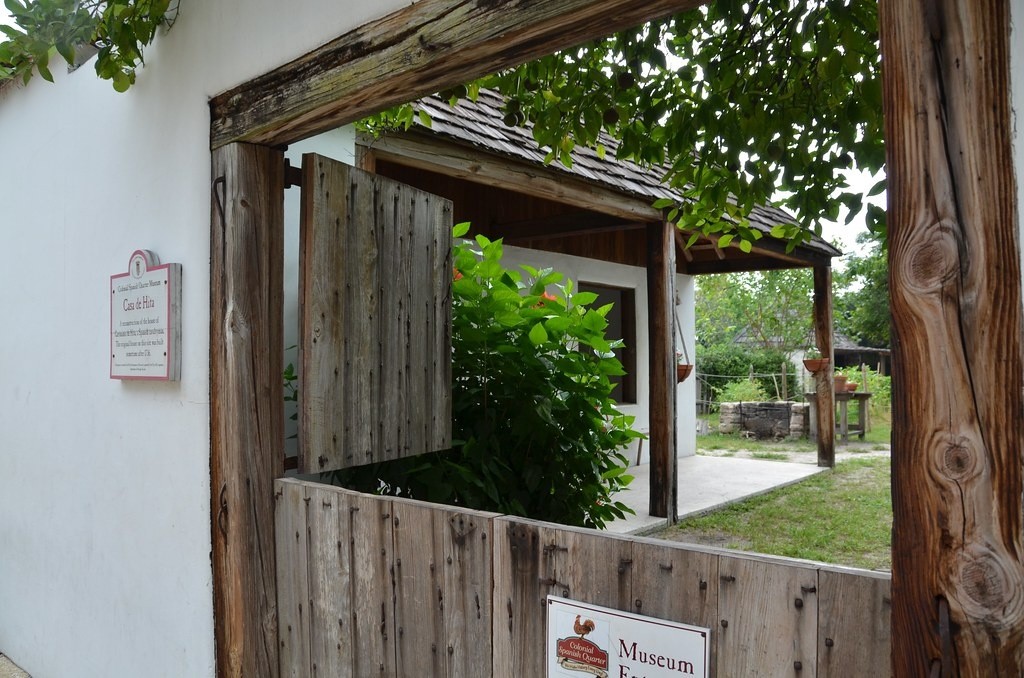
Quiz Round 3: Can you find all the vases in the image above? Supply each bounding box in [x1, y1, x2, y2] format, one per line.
[802, 360, 829, 372]
[834, 377, 848, 393]
[677, 364, 694, 385]
[847, 383, 858, 393]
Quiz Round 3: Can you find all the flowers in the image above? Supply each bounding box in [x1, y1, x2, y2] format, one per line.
[676, 348, 684, 364]
[834, 366, 848, 377]
[806, 346, 823, 359]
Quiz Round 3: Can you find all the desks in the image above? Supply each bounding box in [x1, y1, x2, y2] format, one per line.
[803, 392, 872, 445]
